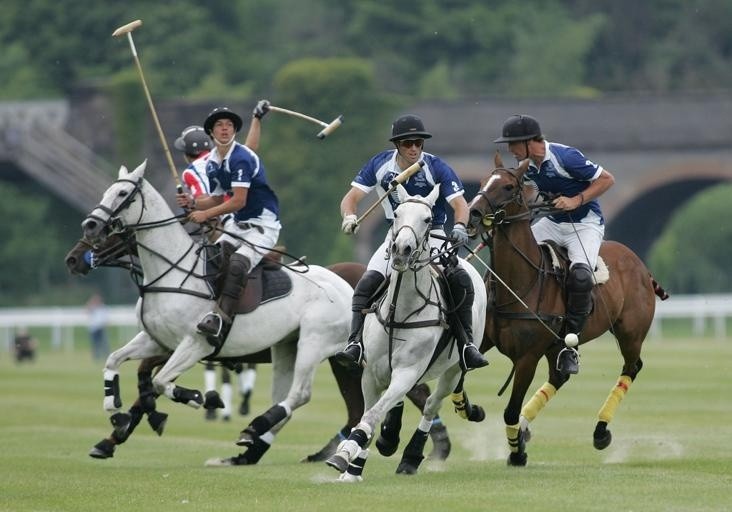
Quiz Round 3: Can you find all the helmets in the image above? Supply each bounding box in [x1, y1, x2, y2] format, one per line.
[204, 106, 243, 135]
[174, 124, 214, 158]
[388, 113, 432, 142]
[492, 113, 542, 145]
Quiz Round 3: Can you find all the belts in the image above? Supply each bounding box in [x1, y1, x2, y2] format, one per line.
[221, 214, 232, 226]
[236, 221, 264, 235]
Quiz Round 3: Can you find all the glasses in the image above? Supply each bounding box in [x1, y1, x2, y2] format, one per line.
[396, 139, 424, 148]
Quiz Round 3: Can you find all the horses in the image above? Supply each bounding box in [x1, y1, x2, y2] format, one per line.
[62, 157, 451, 470]
[450, 150, 671, 468]
[327, 182, 488, 484]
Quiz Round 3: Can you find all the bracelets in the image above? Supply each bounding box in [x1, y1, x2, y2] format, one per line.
[191, 197, 195, 210]
[577, 192, 584, 210]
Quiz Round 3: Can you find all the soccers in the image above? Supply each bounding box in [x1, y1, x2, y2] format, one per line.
[564, 333, 579, 347]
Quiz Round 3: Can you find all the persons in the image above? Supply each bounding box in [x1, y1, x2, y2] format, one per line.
[175, 107, 283, 347]
[86, 293, 108, 360]
[335, 115, 489, 370]
[493, 115, 615, 374]
[174, 100, 270, 242]
[16, 328, 35, 361]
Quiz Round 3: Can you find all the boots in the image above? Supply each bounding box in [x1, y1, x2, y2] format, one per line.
[559, 291, 591, 375]
[334, 295, 376, 374]
[448, 294, 489, 371]
[197, 277, 246, 335]
